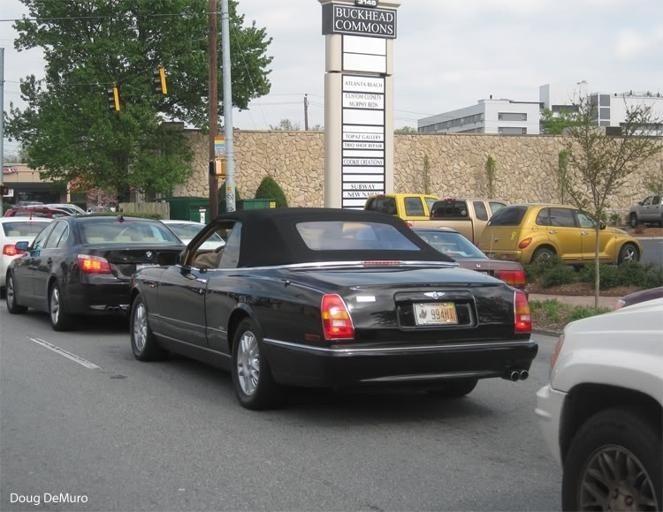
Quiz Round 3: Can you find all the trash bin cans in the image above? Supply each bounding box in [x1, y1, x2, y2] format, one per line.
[236, 198, 279, 210]
[169, 197, 211, 224]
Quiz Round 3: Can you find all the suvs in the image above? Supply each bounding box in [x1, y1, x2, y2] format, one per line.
[521, 292, 662, 512]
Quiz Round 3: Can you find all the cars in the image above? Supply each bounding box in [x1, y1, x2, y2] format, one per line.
[126, 200, 541, 421]
[4, 213, 192, 333]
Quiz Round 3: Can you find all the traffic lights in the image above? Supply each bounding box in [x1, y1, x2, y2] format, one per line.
[106, 88, 121, 113]
[152, 66, 167, 96]
[212, 155, 228, 179]
